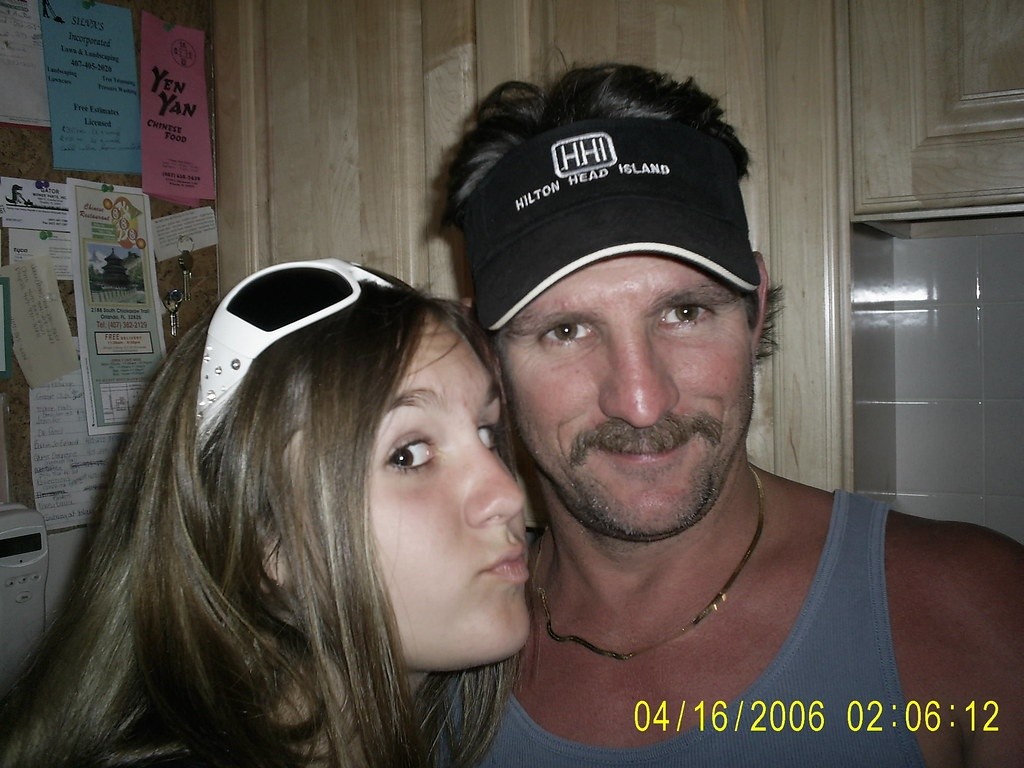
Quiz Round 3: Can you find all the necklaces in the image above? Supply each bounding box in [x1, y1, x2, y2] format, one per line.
[533, 462, 765, 661]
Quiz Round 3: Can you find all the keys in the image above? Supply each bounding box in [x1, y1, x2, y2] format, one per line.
[164, 290, 183, 337]
[178, 250, 193, 303]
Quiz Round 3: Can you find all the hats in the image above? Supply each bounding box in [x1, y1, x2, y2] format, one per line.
[451, 117, 760, 330]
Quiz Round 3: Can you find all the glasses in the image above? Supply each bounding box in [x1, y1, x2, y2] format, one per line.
[192, 258, 423, 477]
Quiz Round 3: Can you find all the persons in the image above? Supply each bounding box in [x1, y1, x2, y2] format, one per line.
[0, 256, 533, 768]
[416, 60, 1024, 768]
